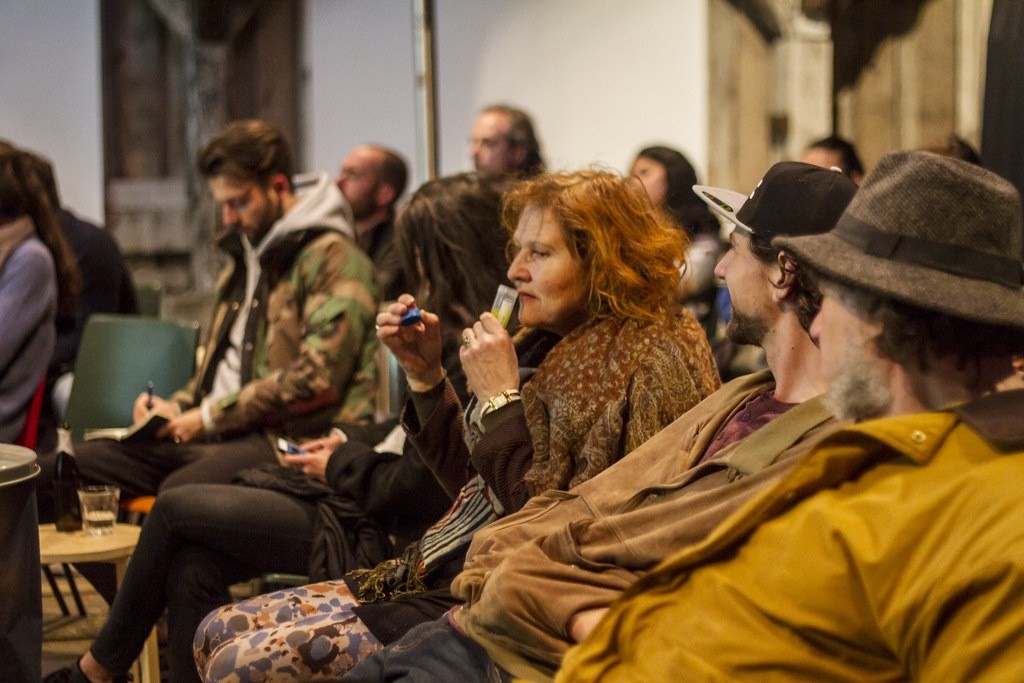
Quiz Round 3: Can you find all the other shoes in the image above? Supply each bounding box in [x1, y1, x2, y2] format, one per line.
[41, 652, 132, 683]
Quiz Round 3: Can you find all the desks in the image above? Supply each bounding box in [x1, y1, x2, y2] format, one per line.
[37, 523, 161, 683]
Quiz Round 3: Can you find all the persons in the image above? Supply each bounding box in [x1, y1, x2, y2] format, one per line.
[801, 136, 865, 188]
[336, 143, 408, 300]
[35, 118, 392, 650]
[190, 161, 725, 683]
[347, 160, 859, 683]
[626, 146, 723, 358]
[0, 137, 138, 456]
[43, 174, 520, 683]
[470, 104, 548, 195]
[548, 148, 1024, 683]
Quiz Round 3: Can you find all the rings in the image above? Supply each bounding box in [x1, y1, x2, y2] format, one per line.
[462, 336, 476, 347]
[374, 323, 381, 330]
[174, 435, 181, 444]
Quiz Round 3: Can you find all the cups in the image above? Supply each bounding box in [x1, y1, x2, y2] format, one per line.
[79, 484, 121, 537]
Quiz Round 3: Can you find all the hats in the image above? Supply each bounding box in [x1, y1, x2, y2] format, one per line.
[771, 148, 1024, 323]
[692, 160, 857, 240]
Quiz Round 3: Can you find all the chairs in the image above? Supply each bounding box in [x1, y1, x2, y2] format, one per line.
[43, 313, 201, 619]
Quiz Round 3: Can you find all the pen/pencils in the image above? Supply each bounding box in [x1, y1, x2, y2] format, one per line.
[146, 381, 153, 410]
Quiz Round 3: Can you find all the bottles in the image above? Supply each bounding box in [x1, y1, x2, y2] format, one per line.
[53, 423, 83, 532]
[491, 284, 519, 330]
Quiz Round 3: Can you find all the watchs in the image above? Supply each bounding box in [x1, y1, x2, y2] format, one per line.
[480, 389, 521, 419]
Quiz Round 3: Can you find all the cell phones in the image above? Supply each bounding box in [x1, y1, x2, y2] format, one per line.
[278, 438, 307, 455]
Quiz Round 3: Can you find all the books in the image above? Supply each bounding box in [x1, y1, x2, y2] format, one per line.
[83, 408, 170, 444]
